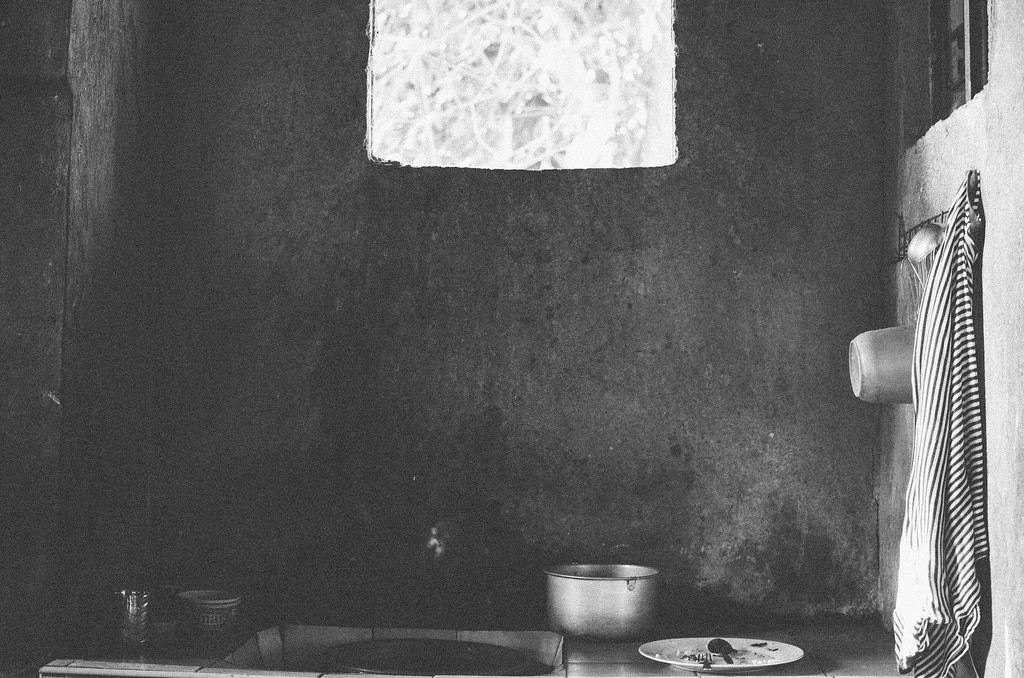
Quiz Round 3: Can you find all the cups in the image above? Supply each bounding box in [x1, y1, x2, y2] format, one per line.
[111, 590, 150, 646]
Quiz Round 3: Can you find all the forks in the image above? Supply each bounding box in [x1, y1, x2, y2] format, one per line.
[698, 653, 713, 671]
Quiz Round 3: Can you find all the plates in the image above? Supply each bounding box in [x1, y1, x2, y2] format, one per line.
[638, 637, 805, 674]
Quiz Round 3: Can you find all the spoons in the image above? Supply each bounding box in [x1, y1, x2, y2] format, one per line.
[707, 638, 734, 664]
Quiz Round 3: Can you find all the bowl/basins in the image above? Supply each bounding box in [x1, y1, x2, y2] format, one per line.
[542, 562, 662, 644]
[178, 589, 243, 639]
